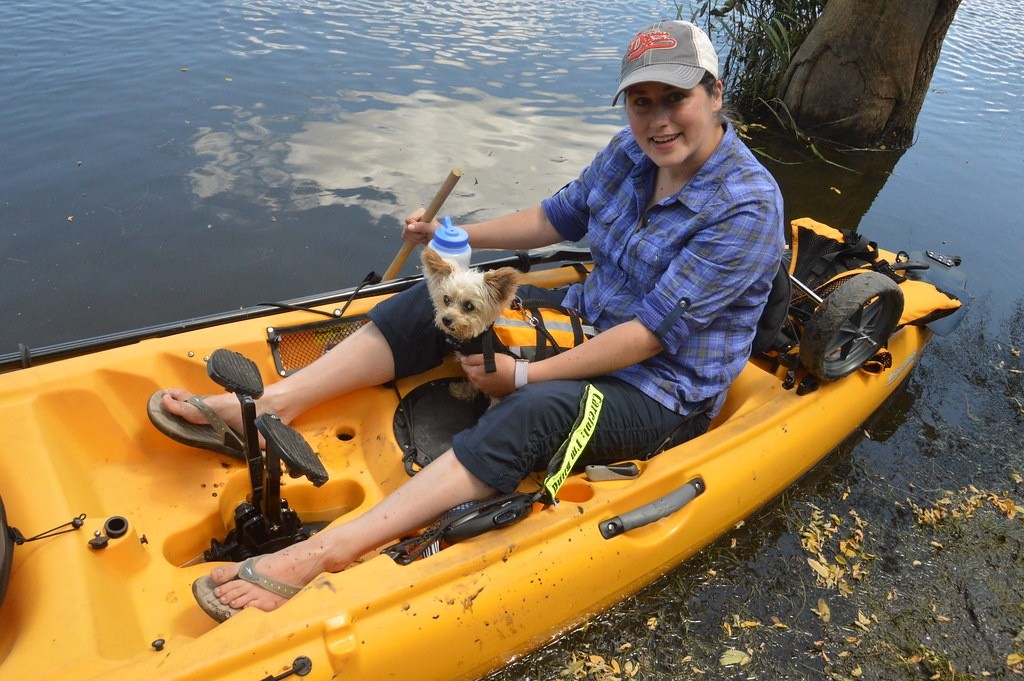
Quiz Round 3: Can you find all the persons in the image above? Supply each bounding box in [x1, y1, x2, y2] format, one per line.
[146, 18, 786, 625]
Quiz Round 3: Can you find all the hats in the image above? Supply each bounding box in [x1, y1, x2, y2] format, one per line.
[611, 19, 719, 107]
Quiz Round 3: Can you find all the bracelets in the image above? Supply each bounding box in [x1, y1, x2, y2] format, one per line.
[513, 358, 529, 389]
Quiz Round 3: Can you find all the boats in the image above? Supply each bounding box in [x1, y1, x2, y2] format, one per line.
[0, 218, 972, 681]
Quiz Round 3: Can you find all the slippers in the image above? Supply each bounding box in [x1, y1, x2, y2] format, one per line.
[191, 553, 305, 625]
[147, 390, 267, 464]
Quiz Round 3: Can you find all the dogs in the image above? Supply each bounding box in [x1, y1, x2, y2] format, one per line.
[420, 249, 602, 411]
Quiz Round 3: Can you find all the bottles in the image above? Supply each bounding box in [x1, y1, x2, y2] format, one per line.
[428, 216, 472, 269]
